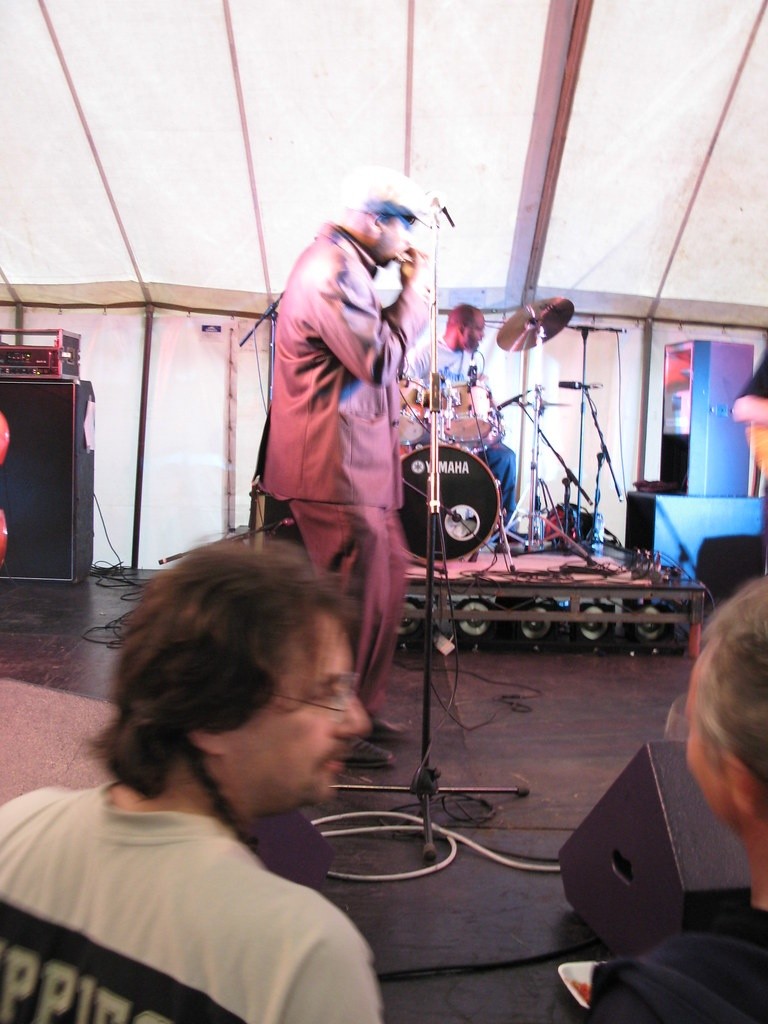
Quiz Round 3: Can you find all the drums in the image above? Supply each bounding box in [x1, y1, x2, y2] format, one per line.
[398, 376, 424, 441]
[401, 442, 502, 562]
[425, 381, 506, 453]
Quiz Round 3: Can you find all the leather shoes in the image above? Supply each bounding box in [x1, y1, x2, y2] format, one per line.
[339, 737, 396, 767]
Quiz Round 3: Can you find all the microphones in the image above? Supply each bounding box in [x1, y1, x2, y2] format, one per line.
[468, 354, 475, 376]
[558, 381, 600, 389]
[450, 513, 461, 522]
[497, 391, 532, 411]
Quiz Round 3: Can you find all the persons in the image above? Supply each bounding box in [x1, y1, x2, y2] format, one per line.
[0, 542, 381, 1024]
[732, 346, 768, 576]
[585, 577, 768, 1024]
[252, 173, 439, 768]
[399, 305, 525, 556]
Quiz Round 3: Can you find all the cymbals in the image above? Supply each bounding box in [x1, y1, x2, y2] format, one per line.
[498, 298, 574, 351]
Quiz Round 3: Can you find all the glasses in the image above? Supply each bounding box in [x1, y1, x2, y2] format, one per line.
[258, 671, 361, 721]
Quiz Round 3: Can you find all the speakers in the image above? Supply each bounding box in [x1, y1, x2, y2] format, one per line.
[624, 491, 768, 623]
[0, 378, 94, 587]
[556, 738, 755, 952]
[657, 339, 754, 498]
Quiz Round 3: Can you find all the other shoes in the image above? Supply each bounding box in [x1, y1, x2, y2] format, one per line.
[495, 538, 525, 555]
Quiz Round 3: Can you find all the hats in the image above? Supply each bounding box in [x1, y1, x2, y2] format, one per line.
[340, 168, 433, 228]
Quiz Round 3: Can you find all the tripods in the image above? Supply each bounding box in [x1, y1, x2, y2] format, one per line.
[331, 193, 530, 862]
[510, 401, 600, 569]
[469, 328, 600, 571]
